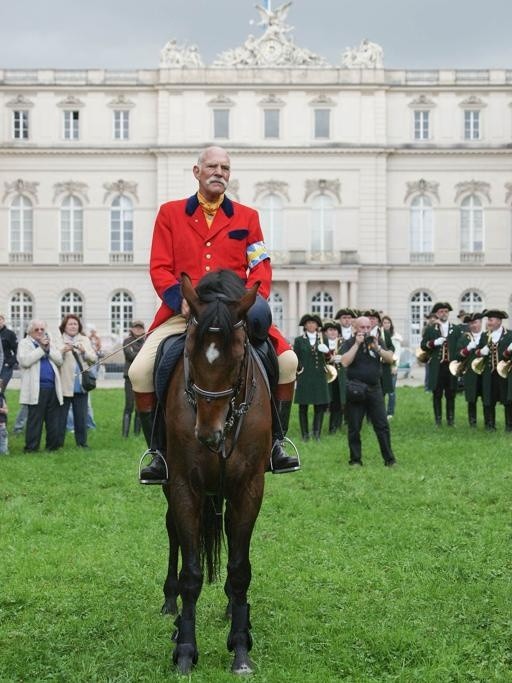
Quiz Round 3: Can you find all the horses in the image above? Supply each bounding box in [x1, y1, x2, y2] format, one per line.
[160, 273, 273, 678]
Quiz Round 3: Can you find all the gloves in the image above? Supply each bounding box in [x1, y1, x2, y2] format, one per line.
[465, 342, 511, 355]
[434, 336, 446, 346]
[318, 343, 342, 365]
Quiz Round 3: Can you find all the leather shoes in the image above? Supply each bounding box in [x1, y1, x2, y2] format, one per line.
[384, 462, 399, 468]
[349, 463, 360, 468]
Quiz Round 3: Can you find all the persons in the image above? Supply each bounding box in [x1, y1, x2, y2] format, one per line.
[0, 314, 145, 455]
[294, 302, 512, 467]
[127, 146, 299, 480]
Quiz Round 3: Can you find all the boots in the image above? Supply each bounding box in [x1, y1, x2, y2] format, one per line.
[433, 398, 511, 434]
[138, 407, 167, 480]
[300, 410, 340, 441]
[266, 398, 299, 472]
[123, 411, 141, 437]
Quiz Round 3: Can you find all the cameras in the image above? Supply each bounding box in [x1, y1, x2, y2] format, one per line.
[362, 333, 374, 344]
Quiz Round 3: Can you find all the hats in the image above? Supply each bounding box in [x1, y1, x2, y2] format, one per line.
[299, 308, 382, 332]
[425, 304, 509, 321]
[132, 321, 144, 328]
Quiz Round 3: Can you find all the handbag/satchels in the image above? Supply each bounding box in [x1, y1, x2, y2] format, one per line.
[344, 379, 369, 404]
[82, 370, 97, 391]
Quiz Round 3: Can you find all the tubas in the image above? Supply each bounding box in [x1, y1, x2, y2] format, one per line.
[417, 318, 439, 362]
[496, 356, 512, 378]
[448, 331, 471, 376]
[471, 329, 492, 375]
[318, 330, 338, 383]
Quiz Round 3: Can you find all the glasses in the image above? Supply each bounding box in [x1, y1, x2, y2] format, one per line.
[30, 328, 45, 333]
